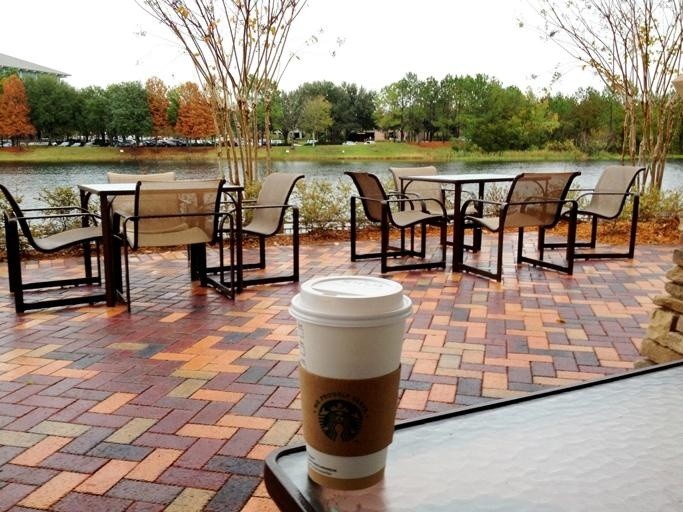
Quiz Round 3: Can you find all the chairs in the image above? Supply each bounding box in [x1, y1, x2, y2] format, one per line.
[540, 165, 647, 260]
[112, 178, 236, 312]
[387, 165, 481, 259]
[0, 185, 105, 314]
[343, 170, 449, 275]
[198, 172, 306, 294]
[106, 170, 192, 263]
[460, 170, 583, 284]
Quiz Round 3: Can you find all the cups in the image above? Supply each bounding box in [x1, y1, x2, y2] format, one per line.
[287, 275, 413, 490]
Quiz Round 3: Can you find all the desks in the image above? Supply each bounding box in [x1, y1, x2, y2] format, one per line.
[398, 172, 517, 275]
[75, 183, 247, 329]
[263, 357, 683, 512]
